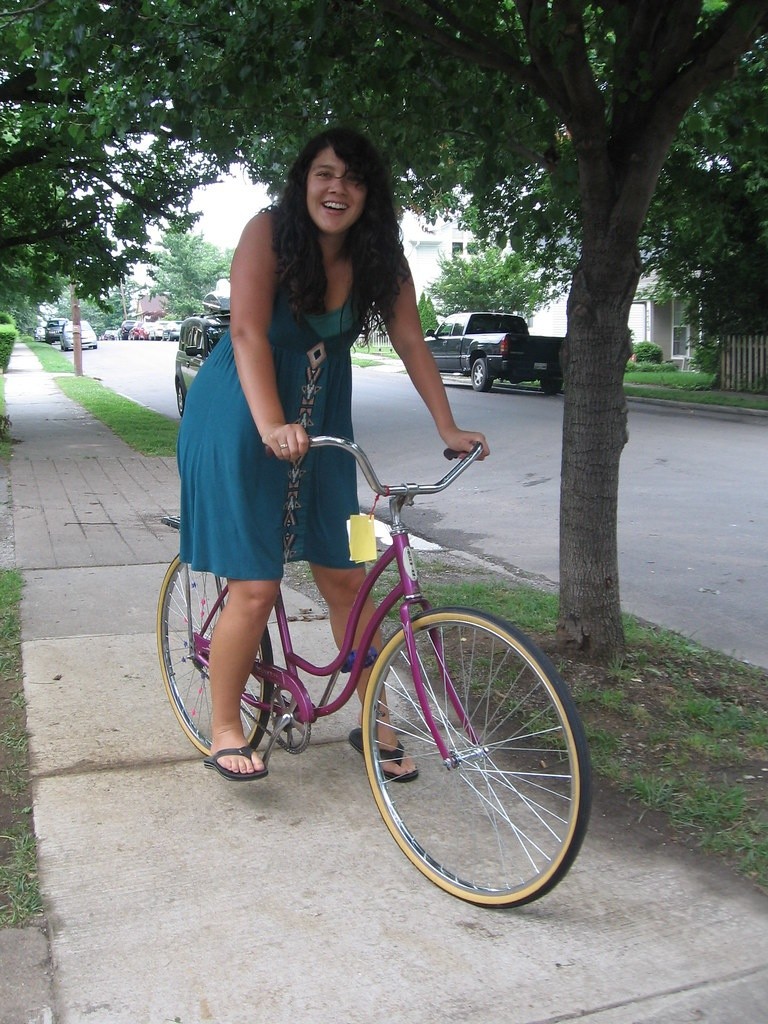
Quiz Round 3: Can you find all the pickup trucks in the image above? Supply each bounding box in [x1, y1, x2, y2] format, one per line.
[423, 312, 567, 394]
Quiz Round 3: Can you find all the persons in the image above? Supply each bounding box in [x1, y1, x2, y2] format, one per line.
[177, 129, 491, 783]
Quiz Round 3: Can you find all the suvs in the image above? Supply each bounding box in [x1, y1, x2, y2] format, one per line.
[174, 292, 231, 417]
[43, 318, 70, 344]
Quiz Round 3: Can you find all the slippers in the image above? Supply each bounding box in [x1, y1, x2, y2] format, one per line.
[203, 744, 269, 782]
[349, 727, 419, 783]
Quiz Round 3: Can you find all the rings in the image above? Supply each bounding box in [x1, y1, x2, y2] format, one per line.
[281, 443, 288, 449]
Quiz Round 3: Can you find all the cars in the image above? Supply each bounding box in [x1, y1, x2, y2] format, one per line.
[59, 321, 98, 351]
[34, 326, 46, 342]
[99, 320, 184, 341]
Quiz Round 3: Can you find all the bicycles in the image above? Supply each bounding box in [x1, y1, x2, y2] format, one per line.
[157, 436, 591, 908]
